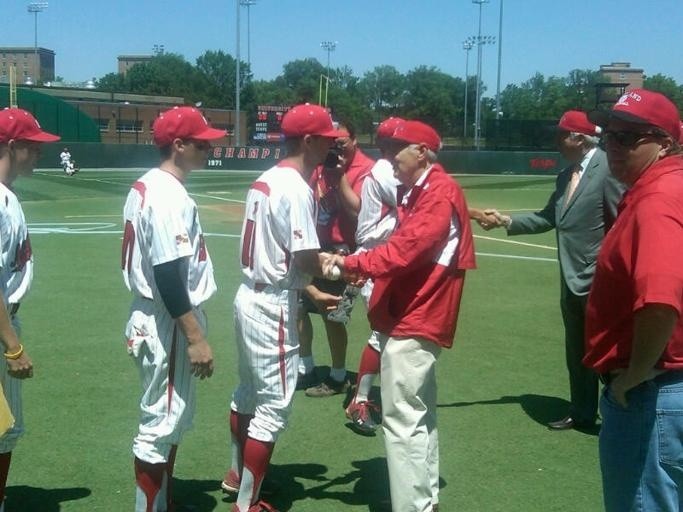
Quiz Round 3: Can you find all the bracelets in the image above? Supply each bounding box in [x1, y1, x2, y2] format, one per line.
[4, 344, 25, 359]
[501, 214, 509, 227]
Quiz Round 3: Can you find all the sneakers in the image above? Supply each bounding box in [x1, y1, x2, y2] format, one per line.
[345, 399, 375, 431]
[222, 469, 281, 495]
[231, 500, 279, 512]
[295, 367, 318, 390]
[305, 371, 351, 397]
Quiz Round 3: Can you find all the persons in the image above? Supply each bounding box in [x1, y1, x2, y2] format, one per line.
[0, 109, 61, 512]
[120, 105, 229, 511]
[581, 89, 683, 510]
[221, 102, 350, 511]
[294, 116, 376, 397]
[59, 148, 78, 177]
[321, 122, 475, 511]
[345, 117, 498, 436]
[481, 110, 627, 430]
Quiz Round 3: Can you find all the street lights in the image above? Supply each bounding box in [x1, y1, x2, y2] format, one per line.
[320, 41, 338, 105]
[241, 0, 256, 80]
[471, 0, 488, 144]
[461, 41, 472, 138]
[468, 36, 496, 138]
[28, 3, 48, 81]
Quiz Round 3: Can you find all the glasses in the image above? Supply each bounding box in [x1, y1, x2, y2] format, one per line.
[604, 130, 667, 145]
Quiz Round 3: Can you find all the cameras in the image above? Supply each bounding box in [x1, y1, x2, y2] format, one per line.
[325, 145, 344, 169]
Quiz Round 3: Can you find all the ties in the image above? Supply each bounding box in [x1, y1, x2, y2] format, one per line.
[567, 165, 580, 206]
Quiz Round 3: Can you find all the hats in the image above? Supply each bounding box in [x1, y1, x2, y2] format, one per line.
[375, 117, 441, 153]
[587, 89, 679, 140]
[281, 102, 350, 138]
[153, 107, 226, 148]
[541, 110, 595, 135]
[0, 107, 60, 143]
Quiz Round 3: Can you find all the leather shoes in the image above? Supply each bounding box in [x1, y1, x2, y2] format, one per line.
[548, 415, 595, 429]
